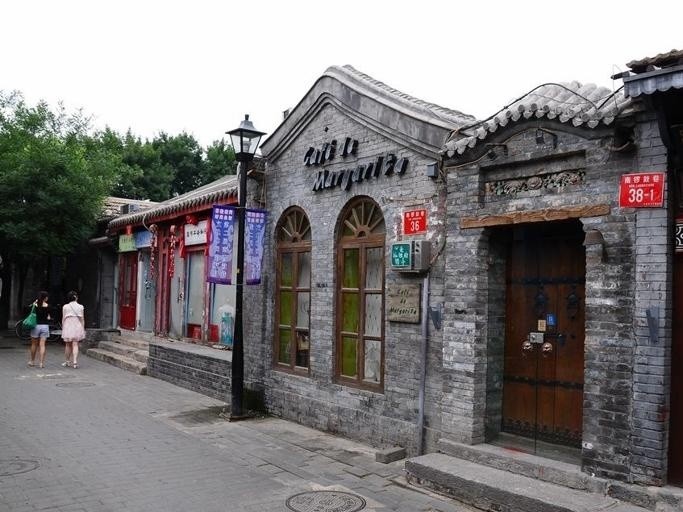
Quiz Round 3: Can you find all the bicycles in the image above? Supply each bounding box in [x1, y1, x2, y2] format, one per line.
[13, 303, 62, 344]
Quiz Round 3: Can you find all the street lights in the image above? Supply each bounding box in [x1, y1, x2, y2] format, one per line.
[224, 114, 267, 421]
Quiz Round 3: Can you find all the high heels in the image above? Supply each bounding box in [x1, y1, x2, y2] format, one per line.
[61, 361, 71, 367]
[73, 362, 80, 369]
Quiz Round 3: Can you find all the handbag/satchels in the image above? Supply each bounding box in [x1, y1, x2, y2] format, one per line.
[22, 303, 37, 330]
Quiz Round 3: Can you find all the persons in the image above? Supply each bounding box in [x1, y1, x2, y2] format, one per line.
[61, 290, 86, 369]
[28, 291, 51, 369]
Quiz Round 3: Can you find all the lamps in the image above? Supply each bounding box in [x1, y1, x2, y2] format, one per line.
[485, 142, 508, 160]
[535, 128, 557, 144]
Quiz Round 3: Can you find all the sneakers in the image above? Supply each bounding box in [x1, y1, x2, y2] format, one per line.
[27, 362, 34, 367]
[39, 364, 46, 369]
